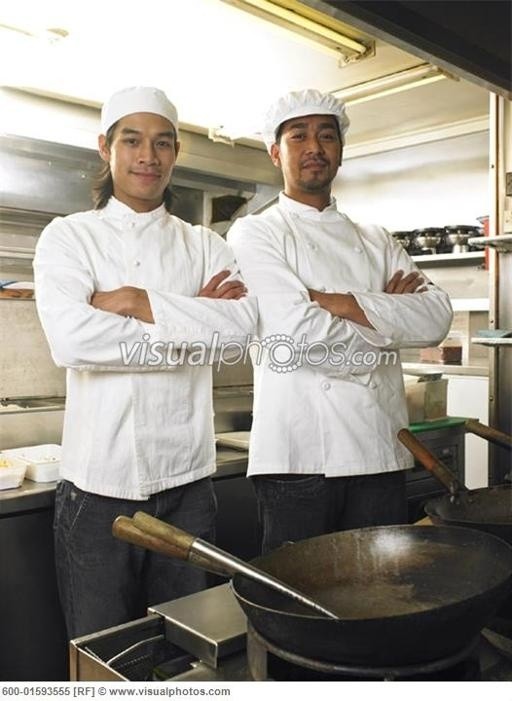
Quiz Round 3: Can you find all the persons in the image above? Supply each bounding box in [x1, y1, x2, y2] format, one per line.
[227, 87, 454, 555]
[29, 84, 259, 641]
[0, 278, 35, 300]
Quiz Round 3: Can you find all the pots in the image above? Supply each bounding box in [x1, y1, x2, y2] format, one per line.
[397, 428, 511, 545]
[111, 515, 512, 670]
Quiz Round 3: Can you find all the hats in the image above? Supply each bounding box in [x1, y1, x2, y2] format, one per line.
[93, 83, 182, 148]
[258, 85, 353, 154]
